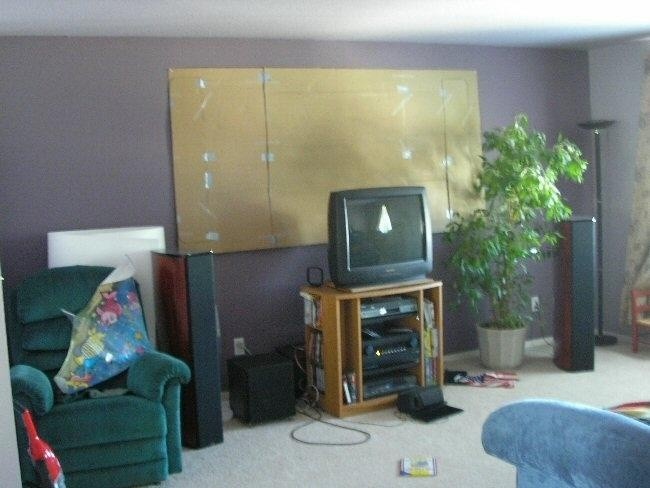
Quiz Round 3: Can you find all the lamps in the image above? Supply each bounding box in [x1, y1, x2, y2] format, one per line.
[578, 117, 616, 346]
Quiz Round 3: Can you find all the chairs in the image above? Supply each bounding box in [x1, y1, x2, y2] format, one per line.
[480, 400, 650, 487]
[3, 266, 191, 488]
[630, 289, 650, 353]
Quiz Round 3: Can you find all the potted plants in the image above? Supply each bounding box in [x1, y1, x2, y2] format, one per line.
[440, 116, 585, 371]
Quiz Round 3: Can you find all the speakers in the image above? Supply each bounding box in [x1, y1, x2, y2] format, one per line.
[226, 351, 294, 425]
[150, 248, 222, 449]
[553, 218, 595, 372]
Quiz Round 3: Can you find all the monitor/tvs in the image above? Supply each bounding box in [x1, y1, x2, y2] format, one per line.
[328, 186, 434, 291]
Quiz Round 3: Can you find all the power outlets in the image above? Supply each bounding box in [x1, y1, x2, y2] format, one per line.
[231, 337, 247, 356]
[530, 296, 541, 313]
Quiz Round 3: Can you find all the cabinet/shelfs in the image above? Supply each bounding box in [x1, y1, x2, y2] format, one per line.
[300, 280, 445, 417]
